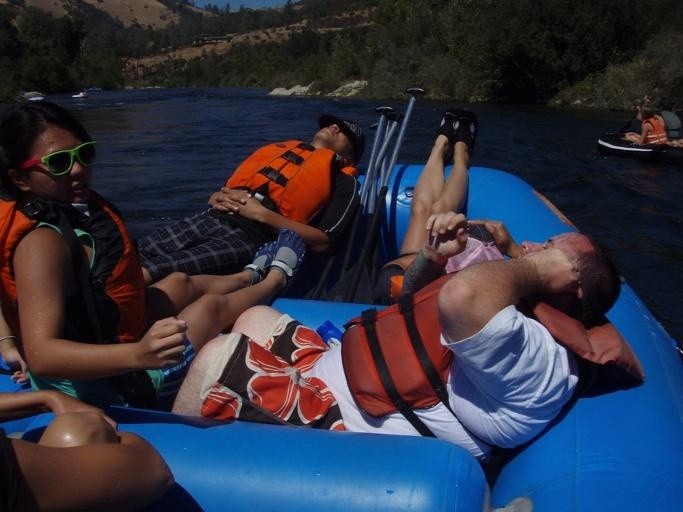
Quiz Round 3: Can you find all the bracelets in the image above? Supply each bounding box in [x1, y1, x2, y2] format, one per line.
[401, 250, 443, 296]
[0, 335, 15, 344]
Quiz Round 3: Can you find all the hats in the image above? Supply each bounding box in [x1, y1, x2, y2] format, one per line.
[443, 223, 503, 274]
[317, 115, 365, 160]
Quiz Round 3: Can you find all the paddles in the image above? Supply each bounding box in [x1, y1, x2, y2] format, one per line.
[301, 86, 428, 304]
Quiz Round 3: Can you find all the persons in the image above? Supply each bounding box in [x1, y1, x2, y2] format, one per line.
[372, 108, 517, 304]
[168, 211, 645, 469]
[0, 389, 533, 511]
[1, 101, 309, 409]
[621, 94, 683, 146]
[0, 313, 29, 385]
[131, 111, 365, 285]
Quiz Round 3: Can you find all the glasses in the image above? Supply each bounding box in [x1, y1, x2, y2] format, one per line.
[19, 140, 98, 176]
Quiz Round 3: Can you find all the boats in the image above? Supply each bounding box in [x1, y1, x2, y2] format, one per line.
[595, 128, 683, 166]
[0, 157, 683, 511]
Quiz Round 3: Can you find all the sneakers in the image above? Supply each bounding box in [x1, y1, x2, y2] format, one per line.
[434, 109, 478, 167]
[243, 228, 307, 294]
